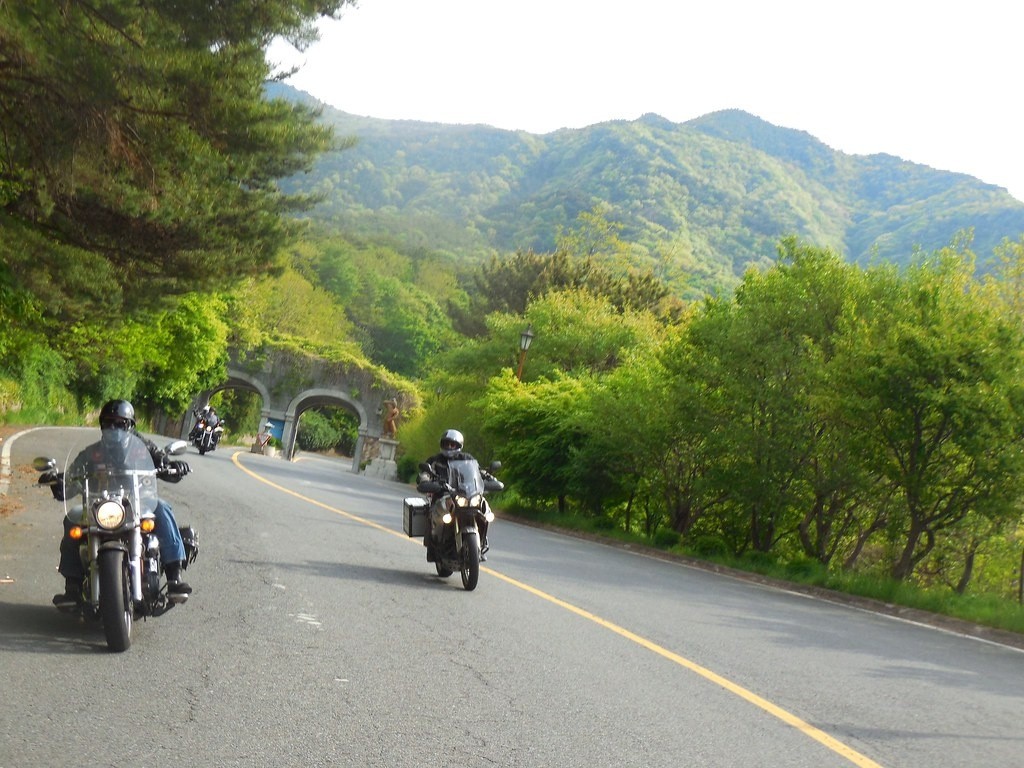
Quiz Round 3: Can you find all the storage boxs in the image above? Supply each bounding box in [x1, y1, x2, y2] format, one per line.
[403, 497, 430, 537]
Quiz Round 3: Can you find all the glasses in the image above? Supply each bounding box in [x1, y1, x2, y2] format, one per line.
[100, 418, 131, 431]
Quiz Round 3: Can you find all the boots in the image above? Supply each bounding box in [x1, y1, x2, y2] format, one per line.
[53, 577, 81, 614]
[165, 566, 192, 593]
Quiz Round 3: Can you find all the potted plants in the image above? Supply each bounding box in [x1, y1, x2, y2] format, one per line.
[359, 462, 367, 476]
[264, 437, 277, 456]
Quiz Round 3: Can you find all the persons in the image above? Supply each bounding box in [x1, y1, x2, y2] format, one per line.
[416, 429, 498, 562]
[189, 406, 220, 449]
[37, 399, 193, 616]
[381, 398, 399, 437]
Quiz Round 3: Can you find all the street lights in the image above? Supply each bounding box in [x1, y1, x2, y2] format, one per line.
[515, 323, 536, 381]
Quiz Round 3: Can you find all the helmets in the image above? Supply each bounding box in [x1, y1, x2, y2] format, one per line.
[209, 408, 216, 412]
[439, 428, 464, 457]
[98, 399, 137, 431]
[203, 406, 210, 411]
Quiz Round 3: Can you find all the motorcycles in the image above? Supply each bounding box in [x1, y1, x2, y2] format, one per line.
[403, 460, 506, 592]
[32, 427, 192, 652]
[194, 414, 226, 454]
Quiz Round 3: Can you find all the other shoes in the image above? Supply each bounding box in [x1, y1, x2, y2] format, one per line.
[430, 528, 437, 549]
[481, 554, 488, 561]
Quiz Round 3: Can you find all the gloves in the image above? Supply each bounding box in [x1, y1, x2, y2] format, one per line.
[38, 473, 63, 491]
[170, 460, 190, 479]
